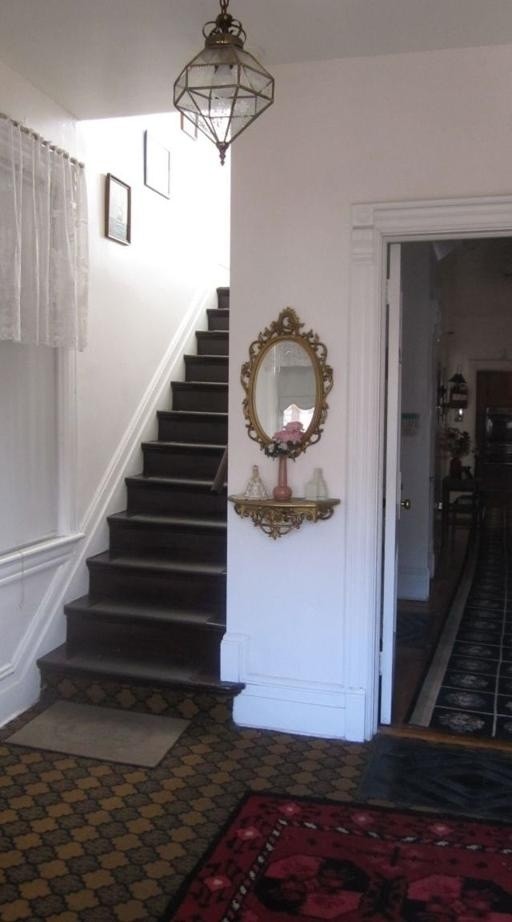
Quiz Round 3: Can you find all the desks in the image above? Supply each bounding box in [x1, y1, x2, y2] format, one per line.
[439, 477, 483, 546]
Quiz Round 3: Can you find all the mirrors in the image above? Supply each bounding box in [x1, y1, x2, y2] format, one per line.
[228, 306, 341, 540]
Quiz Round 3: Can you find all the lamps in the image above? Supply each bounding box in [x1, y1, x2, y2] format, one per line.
[443, 373, 470, 423]
[173, 0, 277, 166]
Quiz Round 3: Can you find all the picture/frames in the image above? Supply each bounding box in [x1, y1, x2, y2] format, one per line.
[104, 172, 133, 247]
[143, 129, 173, 200]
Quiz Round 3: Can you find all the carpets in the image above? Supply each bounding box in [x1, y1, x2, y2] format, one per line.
[5, 699, 192, 768]
[156, 790, 511, 917]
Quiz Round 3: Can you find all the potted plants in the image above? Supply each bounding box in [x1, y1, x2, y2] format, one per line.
[440, 427, 470, 479]
